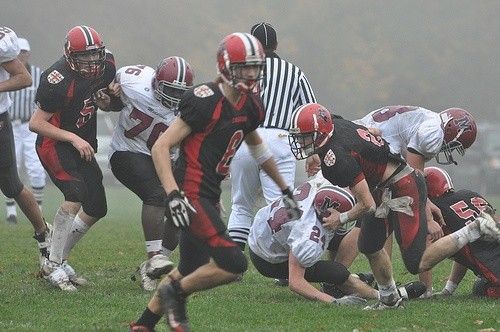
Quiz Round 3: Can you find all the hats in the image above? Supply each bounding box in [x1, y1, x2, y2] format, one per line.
[18, 37, 30, 53]
[252, 23, 276, 47]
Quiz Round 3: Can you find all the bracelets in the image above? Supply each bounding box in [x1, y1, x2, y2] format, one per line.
[340, 211, 353, 225]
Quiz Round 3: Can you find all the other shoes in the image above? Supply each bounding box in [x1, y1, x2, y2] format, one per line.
[6, 215, 17, 225]
[274, 278, 289, 285]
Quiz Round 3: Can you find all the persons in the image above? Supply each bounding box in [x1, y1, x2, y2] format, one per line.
[245, 168, 427, 306]
[227, 22, 321, 286]
[91, 55, 194, 291]
[288, 101, 500, 310]
[29, 26, 121, 294]
[0, 25, 53, 269]
[129, 32, 302, 332]
[5, 38, 48, 226]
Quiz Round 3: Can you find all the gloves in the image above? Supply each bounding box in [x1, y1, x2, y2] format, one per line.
[282, 189, 299, 221]
[333, 295, 367, 306]
[433, 289, 450, 298]
[166, 190, 196, 228]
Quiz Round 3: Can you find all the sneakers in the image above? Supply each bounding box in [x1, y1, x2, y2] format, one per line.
[476, 208, 500, 244]
[33, 221, 53, 269]
[61, 262, 86, 285]
[146, 254, 175, 279]
[131, 321, 155, 332]
[140, 261, 156, 290]
[42, 262, 78, 292]
[363, 300, 405, 311]
[399, 280, 427, 299]
[166, 300, 190, 332]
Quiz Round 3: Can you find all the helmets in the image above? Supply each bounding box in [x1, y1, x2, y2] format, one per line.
[423, 166, 454, 197]
[64, 25, 105, 80]
[218, 32, 266, 93]
[313, 185, 357, 229]
[289, 103, 332, 136]
[156, 56, 193, 111]
[440, 108, 477, 149]
